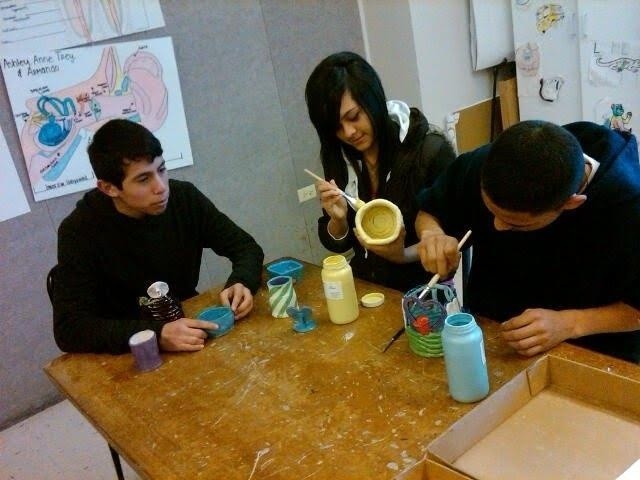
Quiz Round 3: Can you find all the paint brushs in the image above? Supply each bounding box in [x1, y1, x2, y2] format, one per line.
[413, 231, 472, 308]
[303, 170, 365, 213]
[380, 316, 403, 352]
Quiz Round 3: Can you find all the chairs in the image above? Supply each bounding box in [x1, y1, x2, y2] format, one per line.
[444, 230, 479, 315]
[47, 262, 202, 479]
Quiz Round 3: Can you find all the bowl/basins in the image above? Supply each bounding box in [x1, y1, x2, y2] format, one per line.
[197, 305, 234, 337]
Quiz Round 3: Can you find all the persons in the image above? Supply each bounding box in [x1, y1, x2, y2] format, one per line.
[412, 119, 639, 357]
[301, 50, 458, 308]
[51, 118, 265, 356]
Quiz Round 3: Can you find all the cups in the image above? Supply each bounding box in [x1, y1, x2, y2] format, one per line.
[129, 329, 163, 372]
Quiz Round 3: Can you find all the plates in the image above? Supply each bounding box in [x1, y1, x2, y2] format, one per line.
[266, 259, 305, 280]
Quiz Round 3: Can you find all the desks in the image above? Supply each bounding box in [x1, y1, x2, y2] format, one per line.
[44, 257, 639, 479]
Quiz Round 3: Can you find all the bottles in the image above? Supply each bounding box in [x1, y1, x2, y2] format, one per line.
[321, 255, 360, 325]
[267, 275, 300, 319]
[441, 312, 490, 403]
